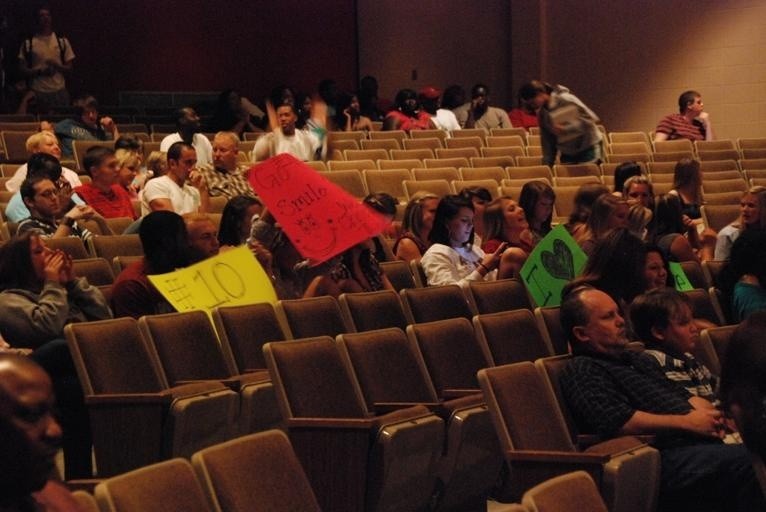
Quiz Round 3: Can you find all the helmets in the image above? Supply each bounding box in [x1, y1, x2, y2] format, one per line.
[419, 87, 442, 111]
[471, 85, 490, 98]
[396, 88, 417, 105]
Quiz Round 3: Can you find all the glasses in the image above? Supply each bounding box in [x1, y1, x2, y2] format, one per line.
[628, 192, 649, 198]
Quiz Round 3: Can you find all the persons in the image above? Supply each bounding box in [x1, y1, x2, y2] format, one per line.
[235, 78, 543, 131]
[516, 79, 606, 167]
[3, 92, 396, 512]
[360, 160, 718, 289]
[14, 6, 77, 111]
[654, 90, 711, 141]
[558, 184, 766, 510]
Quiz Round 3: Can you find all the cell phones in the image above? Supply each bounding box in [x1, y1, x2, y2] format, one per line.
[495, 243, 509, 255]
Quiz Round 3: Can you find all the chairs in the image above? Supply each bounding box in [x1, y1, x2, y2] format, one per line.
[0, 112, 765, 512]
